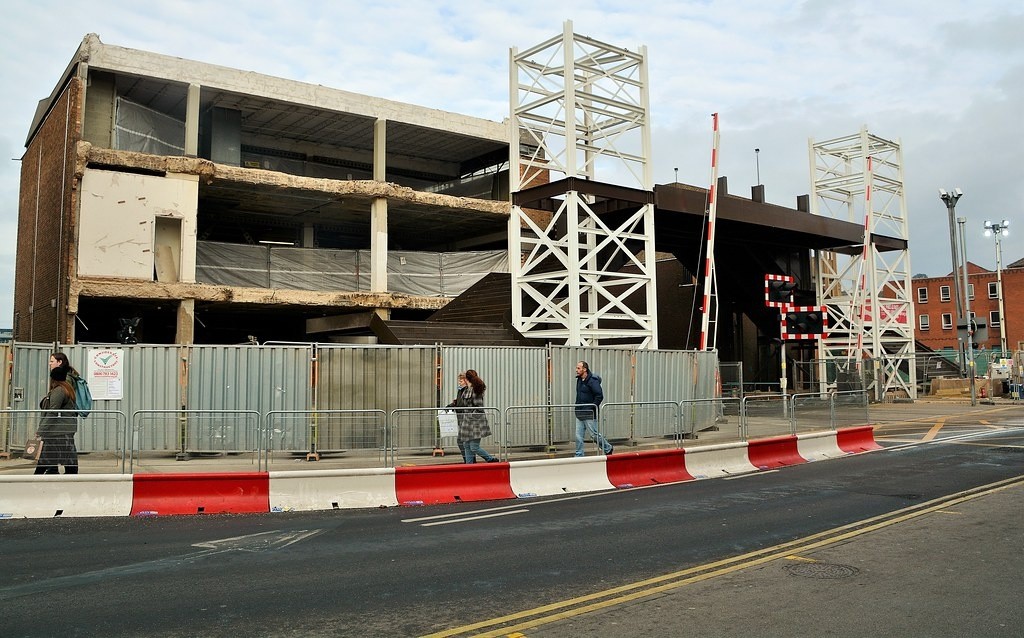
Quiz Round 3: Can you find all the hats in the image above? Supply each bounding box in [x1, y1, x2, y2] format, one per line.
[49, 367, 67, 381]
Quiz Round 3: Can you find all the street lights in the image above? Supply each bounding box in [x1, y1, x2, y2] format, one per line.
[982, 218, 1010, 358]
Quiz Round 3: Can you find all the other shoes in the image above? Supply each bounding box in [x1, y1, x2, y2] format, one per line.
[606, 445, 613, 455]
[487, 458, 499, 462]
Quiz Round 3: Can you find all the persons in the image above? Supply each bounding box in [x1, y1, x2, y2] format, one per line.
[35, 367, 80, 474]
[444, 373, 477, 464]
[573, 360, 614, 458]
[459, 370, 500, 464]
[33, 352, 82, 475]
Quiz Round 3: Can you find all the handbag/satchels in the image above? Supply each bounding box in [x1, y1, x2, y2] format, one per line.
[23, 436, 44, 461]
[438, 409, 459, 438]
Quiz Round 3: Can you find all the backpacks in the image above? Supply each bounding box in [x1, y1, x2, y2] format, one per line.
[66, 373, 92, 420]
